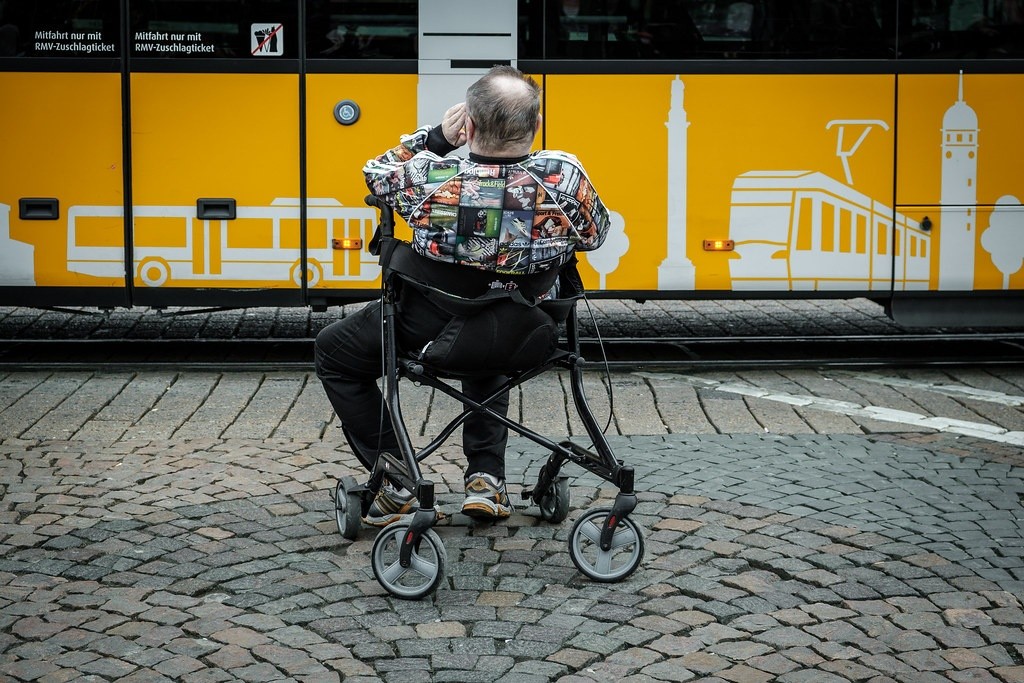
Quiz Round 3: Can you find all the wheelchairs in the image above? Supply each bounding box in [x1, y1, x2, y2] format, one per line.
[333, 193, 649, 598]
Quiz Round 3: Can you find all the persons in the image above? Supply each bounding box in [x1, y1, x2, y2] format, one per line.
[311, 65, 616, 528]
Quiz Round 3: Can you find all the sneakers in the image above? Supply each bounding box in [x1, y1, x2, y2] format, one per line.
[366, 486, 443, 521]
[462, 472, 513, 518]
